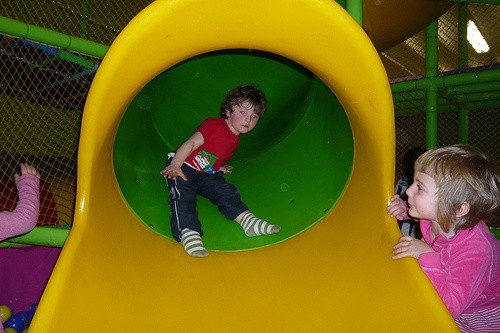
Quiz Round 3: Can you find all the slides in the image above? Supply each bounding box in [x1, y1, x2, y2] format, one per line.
[25, 0, 461, 333]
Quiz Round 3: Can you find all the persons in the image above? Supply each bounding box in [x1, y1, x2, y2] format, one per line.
[0, 163, 41, 333]
[160, 83, 282, 257]
[394, 147, 427, 239]
[387, 144, 500, 333]
